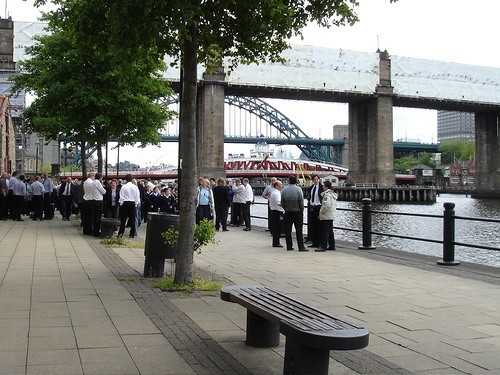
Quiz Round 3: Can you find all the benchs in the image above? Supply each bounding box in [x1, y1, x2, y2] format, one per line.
[220, 285, 369, 375]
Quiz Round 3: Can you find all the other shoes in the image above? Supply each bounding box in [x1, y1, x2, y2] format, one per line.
[326, 246, 336, 250]
[287, 247, 294, 250]
[280, 233, 286, 238]
[215, 229, 220, 231]
[303, 240, 307, 243]
[223, 228, 229, 232]
[308, 243, 319, 248]
[299, 248, 309, 252]
[243, 227, 251, 231]
[272, 244, 284, 247]
[306, 240, 313, 244]
[315, 246, 326, 252]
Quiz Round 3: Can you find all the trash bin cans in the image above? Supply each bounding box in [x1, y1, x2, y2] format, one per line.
[144, 212, 179, 263]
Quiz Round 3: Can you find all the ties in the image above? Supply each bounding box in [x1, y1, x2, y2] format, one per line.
[42, 180, 45, 184]
[311, 185, 316, 202]
[67, 184, 70, 195]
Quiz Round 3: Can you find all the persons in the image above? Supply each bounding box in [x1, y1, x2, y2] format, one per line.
[242, 178, 254, 231]
[0, 171, 27, 221]
[54, 177, 83, 221]
[137, 178, 178, 223]
[262, 177, 286, 247]
[304, 174, 339, 252]
[213, 177, 230, 231]
[82, 173, 106, 237]
[196, 177, 216, 225]
[281, 175, 310, 251]
[116, 174, 141, 238]
[227, 180, 246, 226]
[27, 173, 54, 221]
[103, 178, 123, 234]
